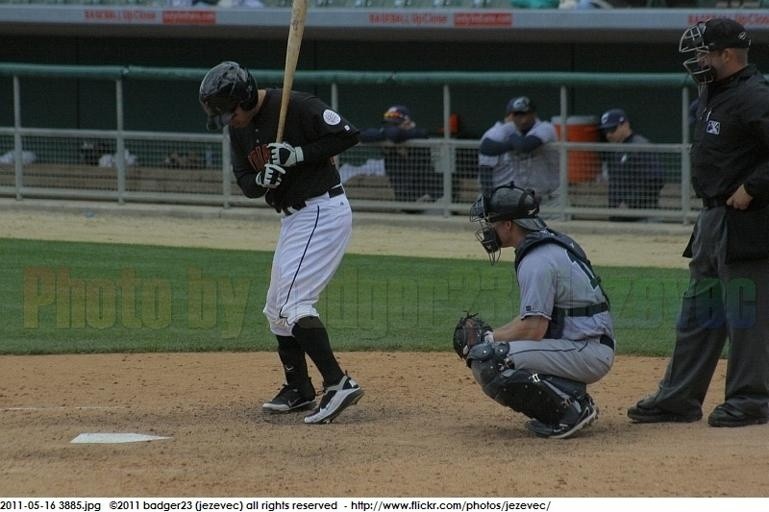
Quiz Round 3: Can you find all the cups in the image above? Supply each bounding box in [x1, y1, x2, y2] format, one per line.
[550, 113, 604, 184]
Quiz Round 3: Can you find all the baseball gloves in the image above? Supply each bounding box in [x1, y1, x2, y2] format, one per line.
[454, 314, 492, 359]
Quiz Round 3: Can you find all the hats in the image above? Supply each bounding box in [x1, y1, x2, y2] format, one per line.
[600, 110, 625, 130]
[510, 95, 535, 115]
[382, 106, 410, 126]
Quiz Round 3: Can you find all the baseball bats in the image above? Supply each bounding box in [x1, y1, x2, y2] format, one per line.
[275, 0, 307, 143]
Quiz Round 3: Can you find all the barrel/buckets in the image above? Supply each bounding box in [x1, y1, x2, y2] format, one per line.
[552, 113, 602, 184]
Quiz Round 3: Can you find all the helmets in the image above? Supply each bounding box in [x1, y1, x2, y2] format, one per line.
[198, 61, 259, 133]
[470, 184, 547, 265]
[677, 17, 753, 97]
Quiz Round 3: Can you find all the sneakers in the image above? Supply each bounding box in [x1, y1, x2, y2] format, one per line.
[261, 376, 316, 414]
[526, 392, 600, 438]
[707, 402, 768, 427]
[303, 371, 363, 425]
[626, 399, 704, 422]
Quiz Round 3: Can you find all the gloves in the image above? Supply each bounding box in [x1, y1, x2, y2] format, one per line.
[266, 142, 304, 168]
[255, 164, 286, 189]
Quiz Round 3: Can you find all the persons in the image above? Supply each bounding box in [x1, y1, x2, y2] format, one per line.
[198, 60, 364, 426]
[478, 97, 516, 194]
[452, 180, 617, 440]
[479, 94, 560, 197]
[359, 105, 444, 217]
[596, 108, 672, 223]
[626, 16, 769, 428]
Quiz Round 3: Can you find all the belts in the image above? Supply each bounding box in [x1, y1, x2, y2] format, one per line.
[599, 333, 615, 350]
[280, 185, 344, 216]
